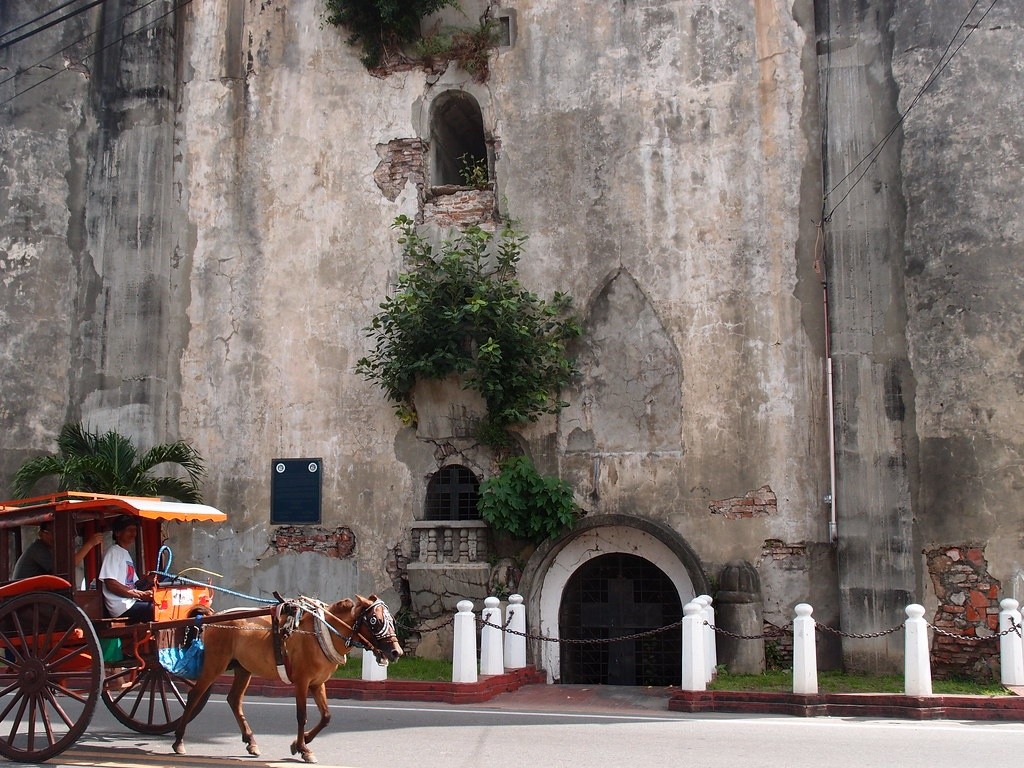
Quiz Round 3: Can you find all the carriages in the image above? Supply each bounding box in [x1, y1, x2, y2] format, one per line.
[0, 492, 402, 765]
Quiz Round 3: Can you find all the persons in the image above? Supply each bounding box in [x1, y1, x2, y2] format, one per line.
[12, 521, 104, 581]
[98, 514, 155, 625]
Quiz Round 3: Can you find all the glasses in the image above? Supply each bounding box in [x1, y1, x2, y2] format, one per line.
[40, 528, 49, 532]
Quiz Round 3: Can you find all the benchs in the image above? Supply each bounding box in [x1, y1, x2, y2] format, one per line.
[80, 617, 129, 629]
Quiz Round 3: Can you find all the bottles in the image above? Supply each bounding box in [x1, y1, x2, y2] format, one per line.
[90, 578, 96, 591]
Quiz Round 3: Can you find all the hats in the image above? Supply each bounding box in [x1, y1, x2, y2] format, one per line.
[112, 515, 137, 540]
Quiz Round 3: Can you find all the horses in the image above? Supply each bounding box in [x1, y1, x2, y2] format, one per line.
[171, 593, 404, 764]
